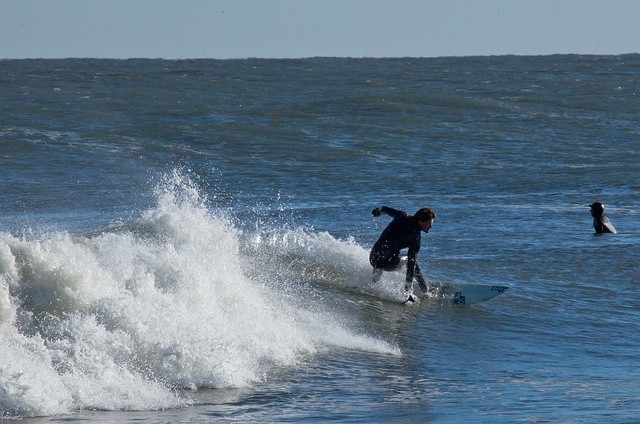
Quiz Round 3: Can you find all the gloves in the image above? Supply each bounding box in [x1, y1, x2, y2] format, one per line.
[372, 207, 380, 217]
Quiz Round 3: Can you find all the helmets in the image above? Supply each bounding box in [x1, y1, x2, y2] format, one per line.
[588, 202, 605, 212]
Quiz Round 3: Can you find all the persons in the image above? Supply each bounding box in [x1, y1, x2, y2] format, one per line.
[589, 201, 617, 234]
[369, 204, 436, 299]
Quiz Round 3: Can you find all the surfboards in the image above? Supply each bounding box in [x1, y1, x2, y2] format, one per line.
[413, 281, 509, 306]
